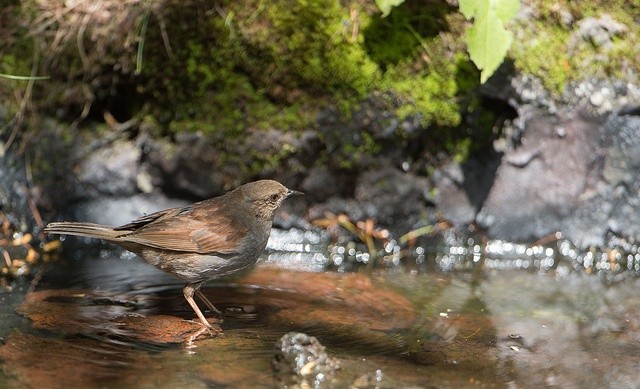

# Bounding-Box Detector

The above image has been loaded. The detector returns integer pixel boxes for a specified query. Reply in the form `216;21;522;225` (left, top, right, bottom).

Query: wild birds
43;178;305;338
